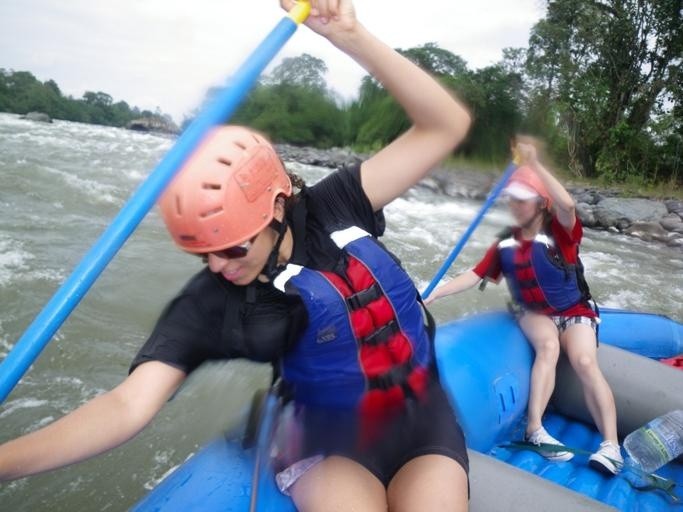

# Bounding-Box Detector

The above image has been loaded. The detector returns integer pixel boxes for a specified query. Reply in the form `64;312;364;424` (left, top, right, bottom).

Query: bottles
623;409;683;476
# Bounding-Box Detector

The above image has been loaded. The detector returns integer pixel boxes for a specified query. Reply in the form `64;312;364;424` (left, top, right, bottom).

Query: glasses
202;236;257;263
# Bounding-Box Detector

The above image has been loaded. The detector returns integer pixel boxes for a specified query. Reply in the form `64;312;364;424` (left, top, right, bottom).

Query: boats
127;307;683;511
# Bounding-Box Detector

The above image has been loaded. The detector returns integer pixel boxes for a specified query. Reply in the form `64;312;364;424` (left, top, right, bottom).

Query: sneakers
523;426;574;462
589;441;624;476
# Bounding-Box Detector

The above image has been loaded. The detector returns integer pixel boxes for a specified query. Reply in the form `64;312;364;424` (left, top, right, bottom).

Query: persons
0;1;474;511
411;130;625;479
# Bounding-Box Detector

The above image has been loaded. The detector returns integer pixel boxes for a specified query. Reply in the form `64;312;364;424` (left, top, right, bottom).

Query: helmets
159;125;293;253
500;165;552;212
